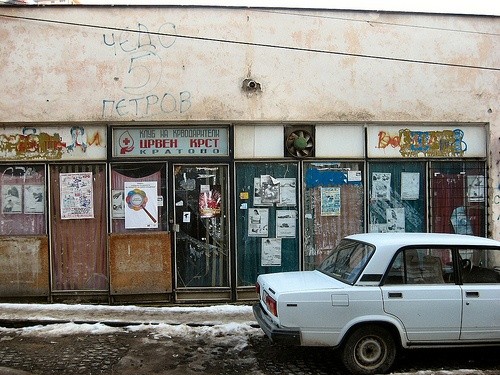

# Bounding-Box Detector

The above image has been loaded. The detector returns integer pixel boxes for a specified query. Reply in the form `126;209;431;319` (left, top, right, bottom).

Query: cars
251;232;499;375
